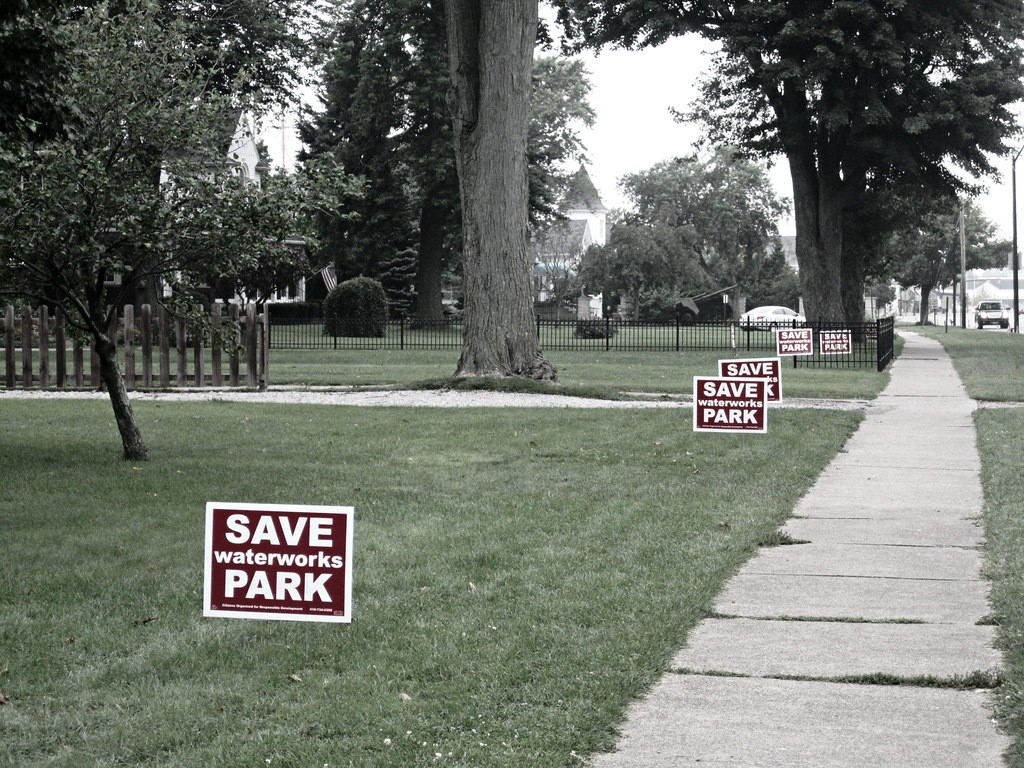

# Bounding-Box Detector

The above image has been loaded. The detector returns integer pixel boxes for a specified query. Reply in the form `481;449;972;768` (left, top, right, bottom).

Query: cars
739;306;806;332
974;301;1010;330
